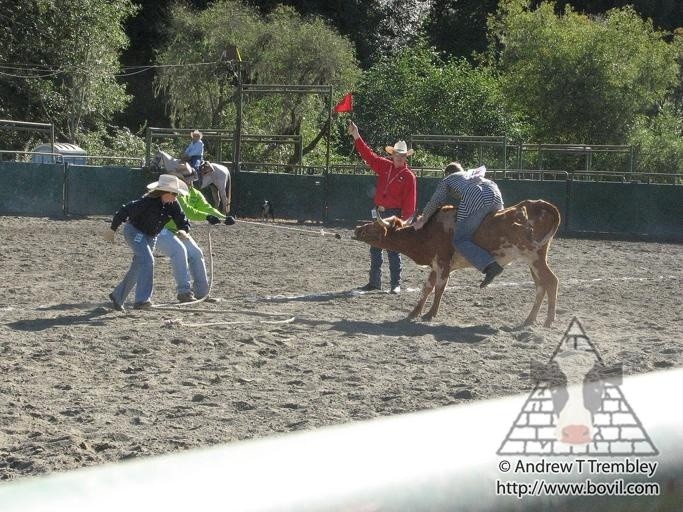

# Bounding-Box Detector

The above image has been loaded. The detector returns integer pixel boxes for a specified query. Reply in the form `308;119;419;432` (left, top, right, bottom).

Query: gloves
206;214;221;225
174;229;187;241
223;215;235;226
101;227;116;245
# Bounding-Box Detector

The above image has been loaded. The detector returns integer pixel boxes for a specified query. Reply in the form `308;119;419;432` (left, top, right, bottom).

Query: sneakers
175;290;197;304
132;301;152;310
107;291;127;315
197;294;219;303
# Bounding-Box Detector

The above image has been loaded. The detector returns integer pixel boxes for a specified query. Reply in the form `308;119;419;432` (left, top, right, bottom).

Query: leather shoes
479;262;504;289
388;285;401;294
356;283;380;291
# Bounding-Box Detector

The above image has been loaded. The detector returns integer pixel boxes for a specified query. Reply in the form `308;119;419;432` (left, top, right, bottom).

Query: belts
375;204;401;212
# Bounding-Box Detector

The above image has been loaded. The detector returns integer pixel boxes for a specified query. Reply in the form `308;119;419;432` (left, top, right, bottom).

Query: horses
148;145;232;216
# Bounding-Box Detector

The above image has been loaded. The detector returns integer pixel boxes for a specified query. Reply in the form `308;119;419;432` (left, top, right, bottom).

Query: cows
530;352;625;447
351;199;561;329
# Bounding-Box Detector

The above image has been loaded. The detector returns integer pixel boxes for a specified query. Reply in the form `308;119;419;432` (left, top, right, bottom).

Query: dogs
260;200;275;223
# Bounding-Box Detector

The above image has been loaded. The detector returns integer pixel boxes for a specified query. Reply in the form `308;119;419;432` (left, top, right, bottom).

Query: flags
331;92;353;112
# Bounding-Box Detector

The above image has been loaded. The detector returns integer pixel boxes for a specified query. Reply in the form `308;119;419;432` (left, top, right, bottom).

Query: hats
383;139;415;157
189;129;202;140
167;161;199;183
145;173;190;197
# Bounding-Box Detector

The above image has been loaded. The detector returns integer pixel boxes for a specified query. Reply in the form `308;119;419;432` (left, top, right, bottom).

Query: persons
181;129;205;189
415;163;504;288
155;163;236;306
108;174;190;311
348;121;417;293
